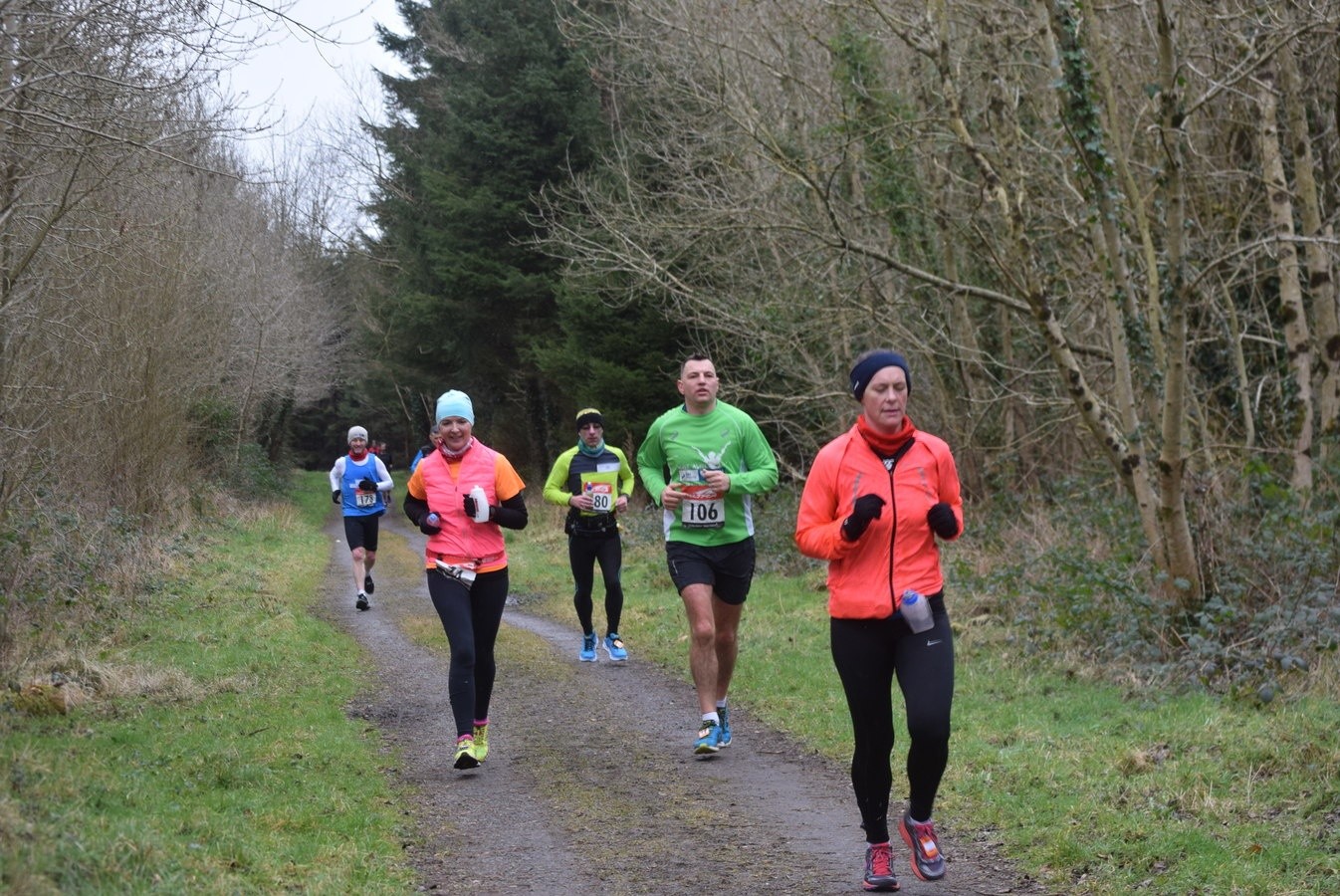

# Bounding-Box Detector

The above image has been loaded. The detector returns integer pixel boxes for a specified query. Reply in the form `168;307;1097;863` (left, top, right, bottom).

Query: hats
436;389;475;430
348;426;369;444
577;408;603;430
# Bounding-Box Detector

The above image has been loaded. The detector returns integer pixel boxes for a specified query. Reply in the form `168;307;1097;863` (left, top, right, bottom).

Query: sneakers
356;595;371;611
898;811;947;881
693;720;722;752
365;576;375;593
579;632;599;663
863;844;900;891
472;721;490;762
453;742;480;769
715;706;732;748
601;632;628;661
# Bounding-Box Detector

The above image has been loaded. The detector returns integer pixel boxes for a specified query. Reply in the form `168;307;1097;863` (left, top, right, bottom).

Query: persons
542;407;634;661
637;353;780;752
795;349;964;890
403;389;529;770
329;425;394;611
367;425;441;507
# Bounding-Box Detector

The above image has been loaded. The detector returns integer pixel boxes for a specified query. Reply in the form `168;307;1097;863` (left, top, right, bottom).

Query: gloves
843;494;887;540
359;476;378;491
463;494;494;521
420;511;442;535
333;489;342;503
926;504;959;539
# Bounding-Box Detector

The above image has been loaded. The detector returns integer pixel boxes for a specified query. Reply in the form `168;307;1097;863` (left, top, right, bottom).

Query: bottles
427;513;441;528
585;482;593;509
900;587;935;634
